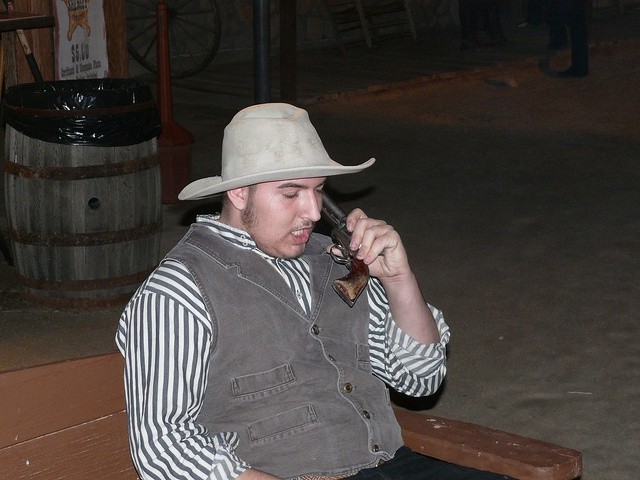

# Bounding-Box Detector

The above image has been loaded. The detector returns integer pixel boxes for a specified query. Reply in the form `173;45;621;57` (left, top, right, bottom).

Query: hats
177;103;376;201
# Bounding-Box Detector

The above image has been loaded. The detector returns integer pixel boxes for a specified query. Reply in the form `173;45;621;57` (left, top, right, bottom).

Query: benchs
0;351;582;479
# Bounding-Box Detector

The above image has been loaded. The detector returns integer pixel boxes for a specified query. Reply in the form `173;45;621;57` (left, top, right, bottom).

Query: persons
115;103;451;480
